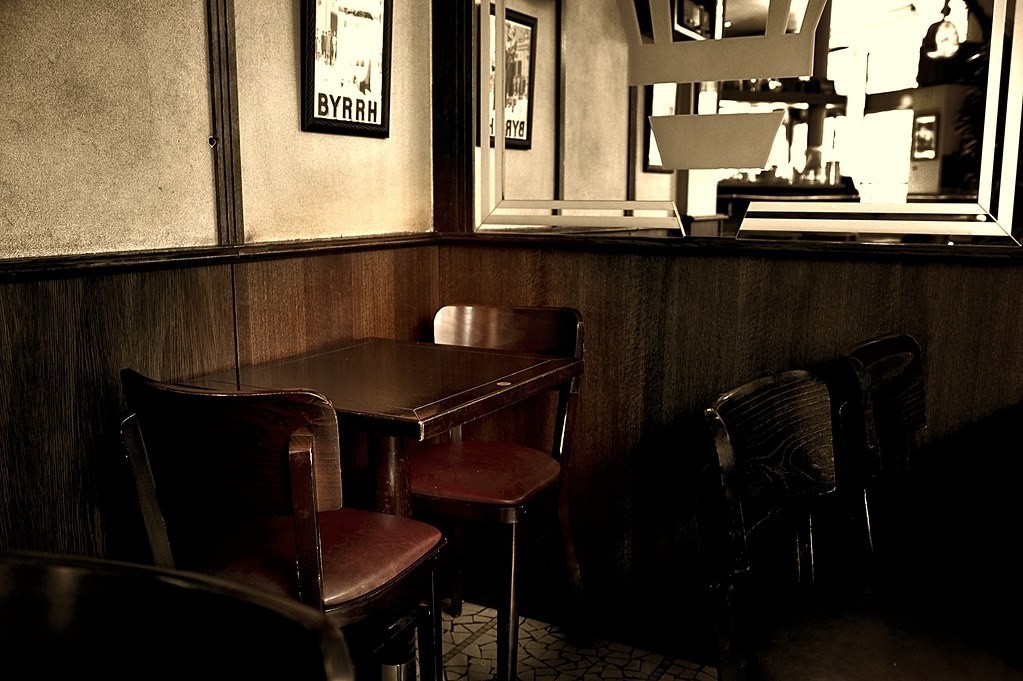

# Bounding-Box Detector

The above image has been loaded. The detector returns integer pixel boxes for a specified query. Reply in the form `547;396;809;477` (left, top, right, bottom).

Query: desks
175;337;586;681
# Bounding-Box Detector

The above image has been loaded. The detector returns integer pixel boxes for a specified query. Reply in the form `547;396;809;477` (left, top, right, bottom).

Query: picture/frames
300;0;393;138
476;2;538;151
910;111;938;161
643;82;679;174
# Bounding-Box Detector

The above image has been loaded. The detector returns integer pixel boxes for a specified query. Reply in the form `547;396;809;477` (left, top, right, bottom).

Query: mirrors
432;0;1023;262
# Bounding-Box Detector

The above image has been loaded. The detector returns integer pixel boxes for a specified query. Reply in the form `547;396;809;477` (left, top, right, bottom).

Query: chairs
0;550;356;681
702;331;1023;681
118;365;450;681
413;305;593;681
